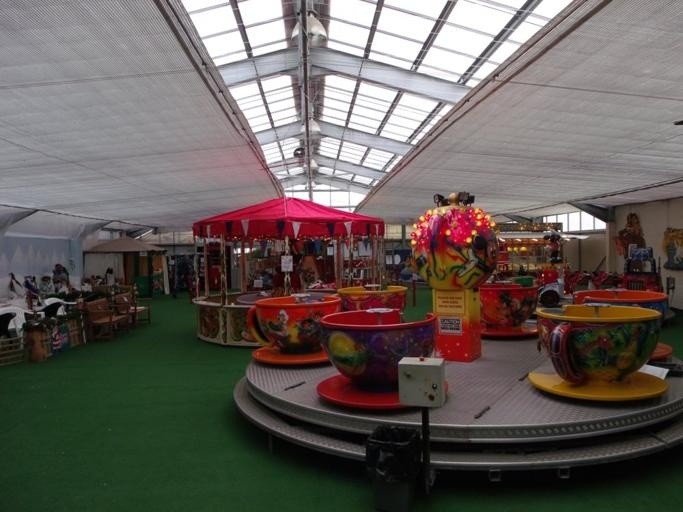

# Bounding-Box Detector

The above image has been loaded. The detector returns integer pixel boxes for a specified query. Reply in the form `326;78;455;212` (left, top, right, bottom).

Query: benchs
85;296;133;342
115;291;151;326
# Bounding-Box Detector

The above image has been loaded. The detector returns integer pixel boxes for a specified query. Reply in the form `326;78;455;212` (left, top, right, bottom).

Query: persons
271;265;283;288
22;262;120;303
618;213;645;257
289;263;301;291
663;226;682;268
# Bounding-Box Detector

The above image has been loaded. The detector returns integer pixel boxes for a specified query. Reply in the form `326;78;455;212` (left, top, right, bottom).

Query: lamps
303;158;318;169
292;0;327;47
306;180;316;188
301;117;320;133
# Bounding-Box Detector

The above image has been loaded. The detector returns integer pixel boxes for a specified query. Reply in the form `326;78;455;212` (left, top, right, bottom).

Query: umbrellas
82;234;167;285
